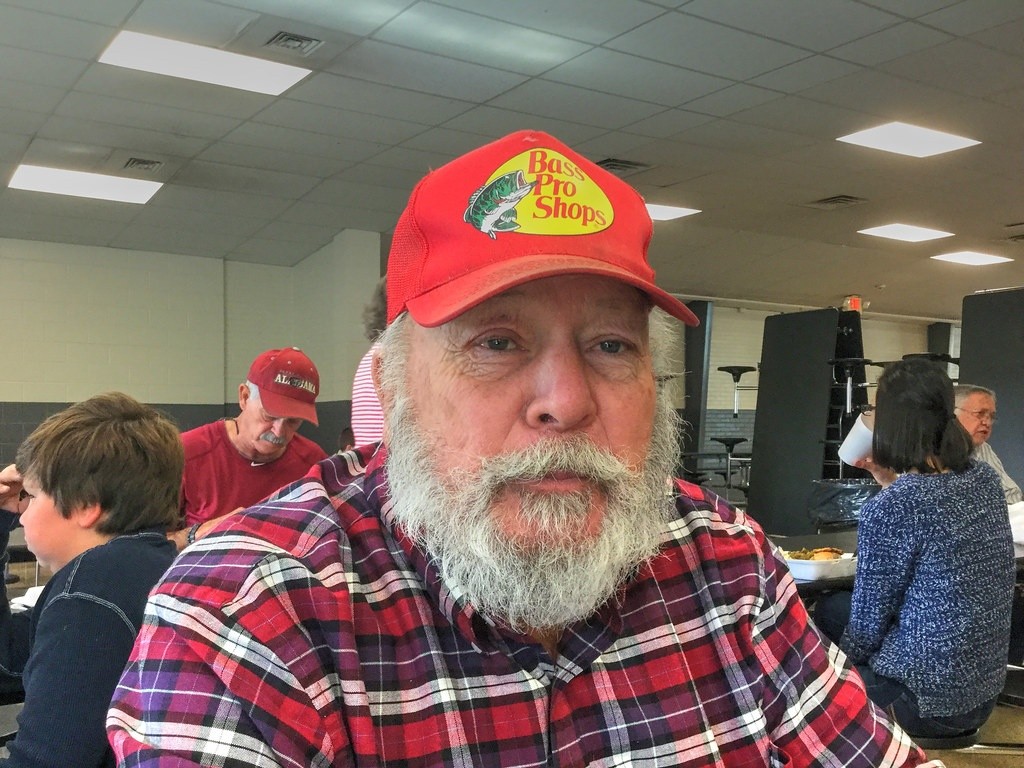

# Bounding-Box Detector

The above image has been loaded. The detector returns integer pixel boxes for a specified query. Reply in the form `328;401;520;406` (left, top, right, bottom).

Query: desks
682;453;752;459
744;287;1024;603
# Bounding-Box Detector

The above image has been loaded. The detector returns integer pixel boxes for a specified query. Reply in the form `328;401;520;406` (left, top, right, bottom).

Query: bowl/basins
777;546;858;580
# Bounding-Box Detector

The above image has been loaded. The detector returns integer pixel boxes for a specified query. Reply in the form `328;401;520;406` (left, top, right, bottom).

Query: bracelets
187;522;203;545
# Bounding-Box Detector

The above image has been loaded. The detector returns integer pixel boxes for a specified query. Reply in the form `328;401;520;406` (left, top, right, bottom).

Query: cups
838;410;875;467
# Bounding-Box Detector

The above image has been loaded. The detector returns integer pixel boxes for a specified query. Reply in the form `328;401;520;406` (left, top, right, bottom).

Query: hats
248;345;323;427
385;129;700;331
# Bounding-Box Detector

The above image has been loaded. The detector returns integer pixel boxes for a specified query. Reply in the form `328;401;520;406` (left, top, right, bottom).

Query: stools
910;664;1024;755
678;352;960;506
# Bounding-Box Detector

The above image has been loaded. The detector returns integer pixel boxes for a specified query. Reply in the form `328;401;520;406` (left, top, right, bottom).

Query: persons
0;391;184;768
351;273;388;449
814;358;1016;738
167;347;329;553
953;384;1023;504
105;129;947;768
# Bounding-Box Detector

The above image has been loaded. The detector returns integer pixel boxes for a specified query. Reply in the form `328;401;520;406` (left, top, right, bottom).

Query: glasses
955;407;999;424
861;404;878;416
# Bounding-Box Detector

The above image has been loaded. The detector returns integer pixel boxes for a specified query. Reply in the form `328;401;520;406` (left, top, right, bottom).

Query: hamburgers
808;547;842;563
